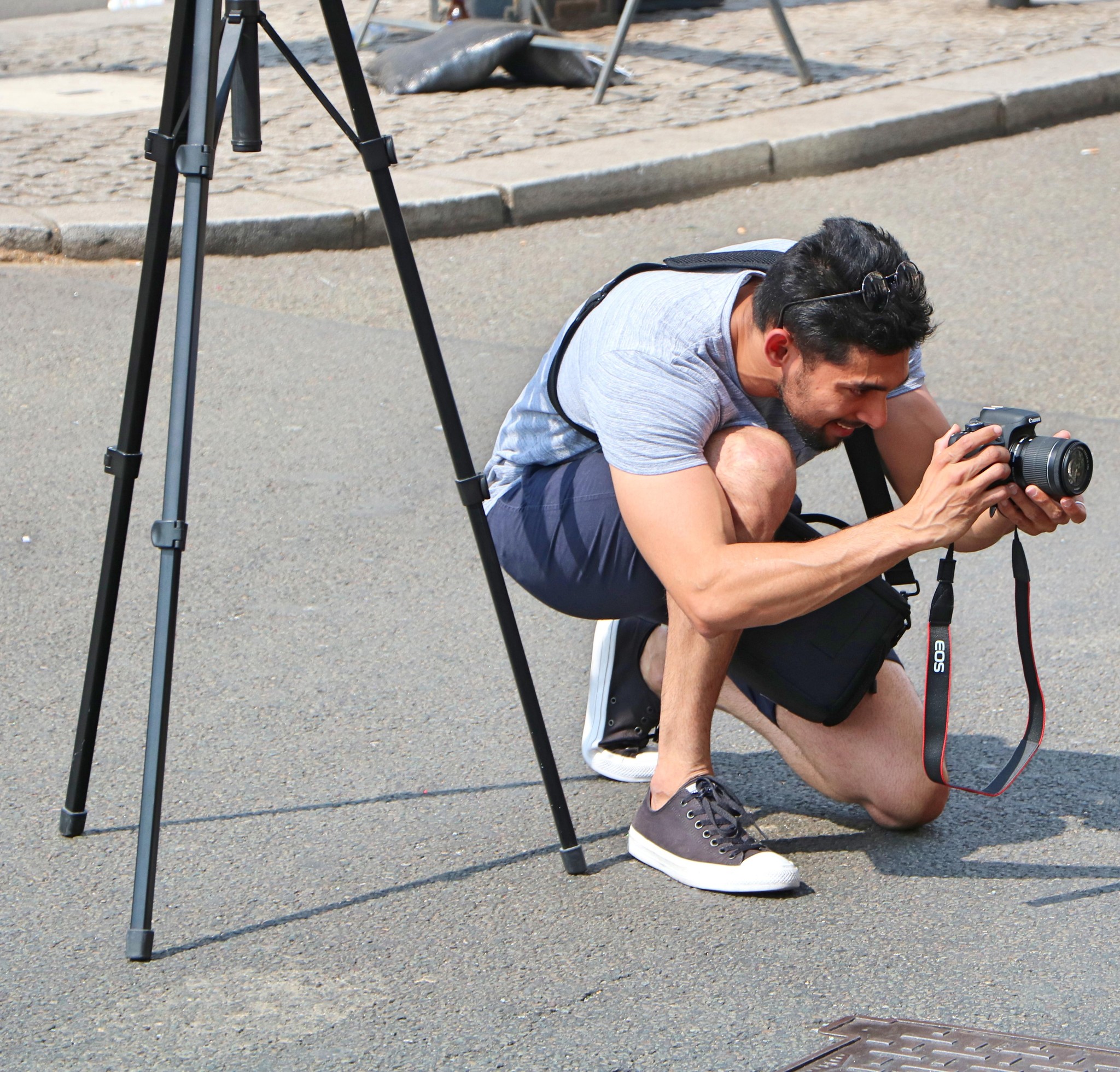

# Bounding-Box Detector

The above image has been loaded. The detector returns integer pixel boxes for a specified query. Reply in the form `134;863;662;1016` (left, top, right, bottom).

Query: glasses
777;262;921;332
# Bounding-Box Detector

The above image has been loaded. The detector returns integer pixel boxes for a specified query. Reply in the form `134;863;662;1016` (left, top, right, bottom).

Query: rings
1075;500;1086;510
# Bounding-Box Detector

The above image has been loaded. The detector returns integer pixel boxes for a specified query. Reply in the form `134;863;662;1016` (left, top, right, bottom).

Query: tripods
58;0;591;961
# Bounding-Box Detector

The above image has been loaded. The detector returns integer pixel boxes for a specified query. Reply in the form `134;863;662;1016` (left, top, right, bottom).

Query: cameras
948;405;1093;503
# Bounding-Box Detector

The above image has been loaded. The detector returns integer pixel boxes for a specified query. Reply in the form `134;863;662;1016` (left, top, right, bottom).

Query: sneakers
582;611;662;780
628;776;799;892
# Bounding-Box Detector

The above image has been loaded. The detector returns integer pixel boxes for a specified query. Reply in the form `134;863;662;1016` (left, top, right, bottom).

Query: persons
478;215;1090;894
